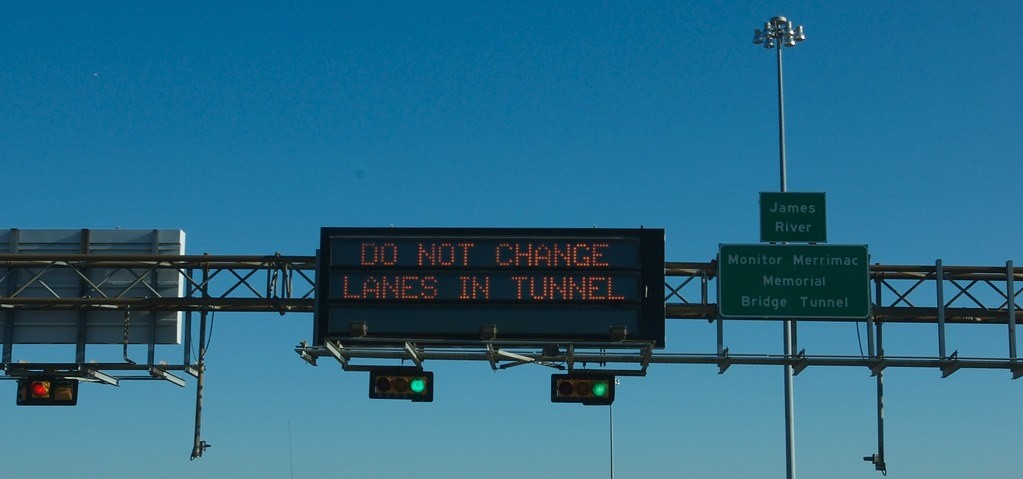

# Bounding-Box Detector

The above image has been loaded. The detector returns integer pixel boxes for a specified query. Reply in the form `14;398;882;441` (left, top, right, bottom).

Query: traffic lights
16;378;78;405
556;378;611;397
373;374;428;396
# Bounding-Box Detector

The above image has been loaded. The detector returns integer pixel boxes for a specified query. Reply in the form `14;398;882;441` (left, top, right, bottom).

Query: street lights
753;13;808;478
